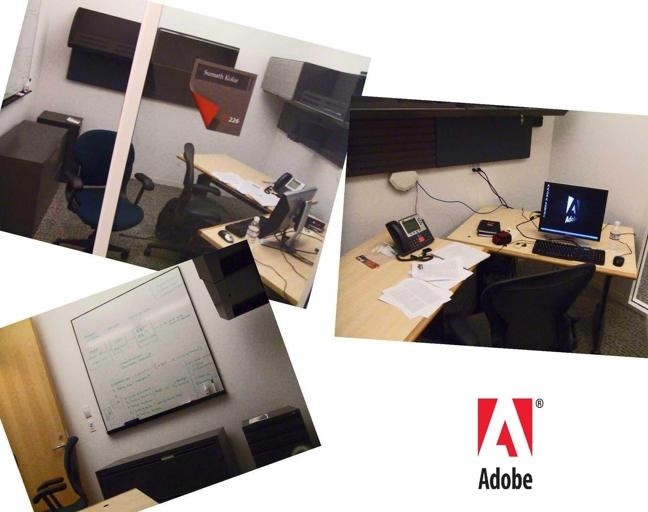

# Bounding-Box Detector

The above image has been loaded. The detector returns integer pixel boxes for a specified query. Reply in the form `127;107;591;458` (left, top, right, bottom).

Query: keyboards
225;215;265;236
533;239;606;265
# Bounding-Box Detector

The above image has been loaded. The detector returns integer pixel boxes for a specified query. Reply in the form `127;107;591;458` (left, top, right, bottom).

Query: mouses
218;230;233;242
612;255;624;267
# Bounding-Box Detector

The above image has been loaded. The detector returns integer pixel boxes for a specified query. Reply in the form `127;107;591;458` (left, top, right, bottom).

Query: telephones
385;214;434;257
272;172;306;195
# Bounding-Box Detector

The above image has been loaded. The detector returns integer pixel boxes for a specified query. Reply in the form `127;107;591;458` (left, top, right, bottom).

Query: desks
173;150;327;307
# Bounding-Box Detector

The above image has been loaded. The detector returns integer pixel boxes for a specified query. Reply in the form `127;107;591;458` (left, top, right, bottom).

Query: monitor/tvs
538;181;608;247
258;187;319;264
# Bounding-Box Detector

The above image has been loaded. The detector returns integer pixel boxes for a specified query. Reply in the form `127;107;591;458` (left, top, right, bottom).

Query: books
477;219;500;238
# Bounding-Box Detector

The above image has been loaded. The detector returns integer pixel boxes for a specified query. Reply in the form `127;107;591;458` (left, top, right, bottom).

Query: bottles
607;221;622;255
245;217;260;251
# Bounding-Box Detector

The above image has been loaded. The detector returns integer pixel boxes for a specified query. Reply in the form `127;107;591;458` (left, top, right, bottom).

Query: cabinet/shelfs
95;406;314;504
0;109;84;238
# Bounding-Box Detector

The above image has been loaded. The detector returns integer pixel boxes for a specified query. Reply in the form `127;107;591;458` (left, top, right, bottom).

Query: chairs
30;434;90;512
145;142;228;266
52;127;155;262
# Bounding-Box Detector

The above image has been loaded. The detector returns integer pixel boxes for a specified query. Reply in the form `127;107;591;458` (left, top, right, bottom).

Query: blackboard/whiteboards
70;266;226;435
0;0;42;108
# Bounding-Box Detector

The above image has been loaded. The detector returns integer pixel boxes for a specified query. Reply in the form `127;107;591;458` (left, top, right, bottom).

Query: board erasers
125;418;139;426
